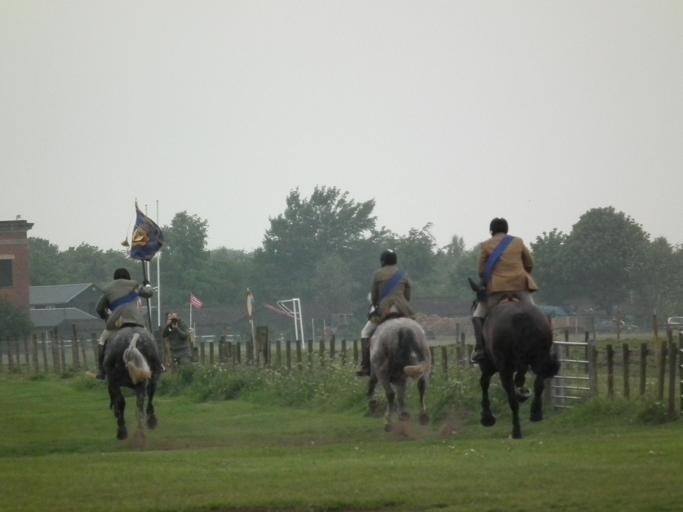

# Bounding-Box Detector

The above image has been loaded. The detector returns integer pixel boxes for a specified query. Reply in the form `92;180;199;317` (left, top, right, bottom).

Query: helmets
114;268;130;280
490;218;508;233
380;249;397;264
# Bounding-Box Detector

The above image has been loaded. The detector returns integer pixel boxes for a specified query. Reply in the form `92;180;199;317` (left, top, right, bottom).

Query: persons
95;266;166;382
162;311;193;382
469;217;539;367
351;248;415;376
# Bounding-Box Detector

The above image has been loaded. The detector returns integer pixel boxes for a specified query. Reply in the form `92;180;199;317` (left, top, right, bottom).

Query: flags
191;295;202;310
129;211;165;261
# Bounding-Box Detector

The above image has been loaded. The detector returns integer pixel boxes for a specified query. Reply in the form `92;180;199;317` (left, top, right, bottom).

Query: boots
355;338;370;376
471;314;486;363
95;344;105;379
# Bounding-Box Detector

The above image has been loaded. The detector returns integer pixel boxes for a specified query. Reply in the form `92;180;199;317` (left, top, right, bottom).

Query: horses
362;292;432;433
102;325;158;440
467;276;561;439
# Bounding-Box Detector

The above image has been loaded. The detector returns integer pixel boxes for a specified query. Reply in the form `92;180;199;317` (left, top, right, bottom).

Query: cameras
171;318;177;325
